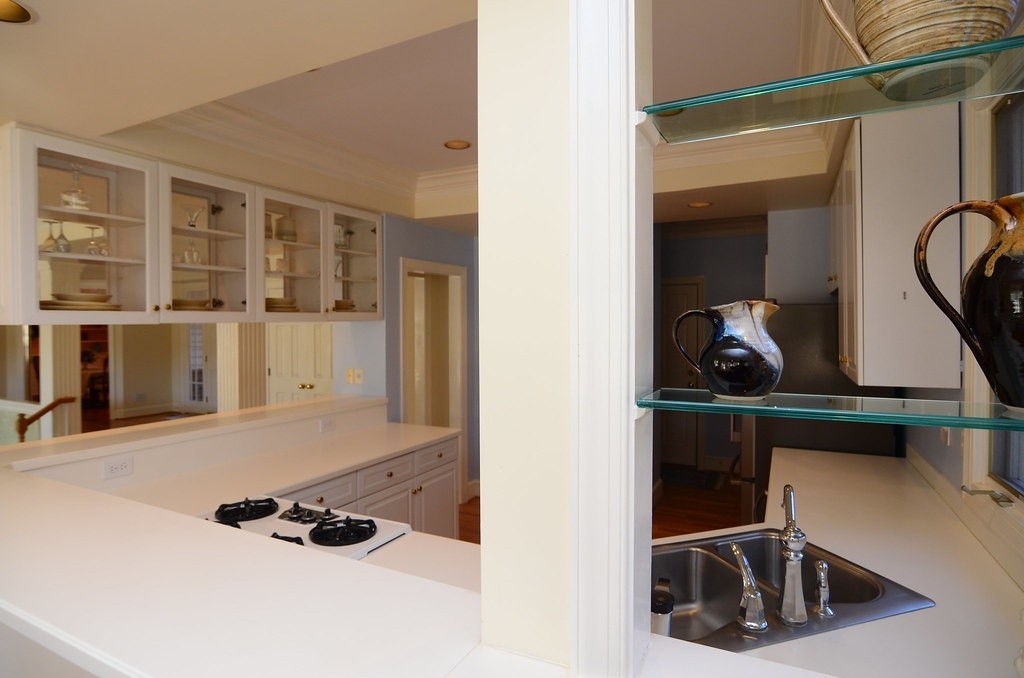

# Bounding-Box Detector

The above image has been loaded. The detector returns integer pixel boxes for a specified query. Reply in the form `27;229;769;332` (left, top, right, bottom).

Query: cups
180;204;206;226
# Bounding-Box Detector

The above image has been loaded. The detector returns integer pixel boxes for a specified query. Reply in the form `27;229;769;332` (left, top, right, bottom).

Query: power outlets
354;368;365;385
345;368;353;384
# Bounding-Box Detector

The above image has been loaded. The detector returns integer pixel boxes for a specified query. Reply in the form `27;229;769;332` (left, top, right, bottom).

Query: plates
40;301;122;312
335;307;355;309
173;305;214;310
266;305;297;308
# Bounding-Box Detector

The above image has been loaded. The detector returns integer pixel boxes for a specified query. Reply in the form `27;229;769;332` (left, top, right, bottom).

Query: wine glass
86;226;113;256
53;218;71;252
40;219;60;252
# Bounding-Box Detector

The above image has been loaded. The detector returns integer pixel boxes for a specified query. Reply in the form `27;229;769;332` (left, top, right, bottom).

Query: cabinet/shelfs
279;472;357;514
18;123;253;324
357;435;460;539
254;188;383;322
827;101;960;386
637;37;1024;432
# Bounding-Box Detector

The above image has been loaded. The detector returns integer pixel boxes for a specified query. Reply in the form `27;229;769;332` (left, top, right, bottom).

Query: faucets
779;484;807;627
728;541;769;632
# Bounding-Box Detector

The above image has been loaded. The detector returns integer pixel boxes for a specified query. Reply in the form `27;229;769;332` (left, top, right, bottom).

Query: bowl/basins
335;299;353;307
265;298;286;304
173;298;210;306
50;293;113;302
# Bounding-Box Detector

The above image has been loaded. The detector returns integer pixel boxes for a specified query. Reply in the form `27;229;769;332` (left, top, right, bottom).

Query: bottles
819;0;1020;101
184;238;201;263
913;194;1024;413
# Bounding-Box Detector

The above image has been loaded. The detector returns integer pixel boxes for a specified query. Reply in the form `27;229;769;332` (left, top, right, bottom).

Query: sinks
650;548;782;652
711;539;887;607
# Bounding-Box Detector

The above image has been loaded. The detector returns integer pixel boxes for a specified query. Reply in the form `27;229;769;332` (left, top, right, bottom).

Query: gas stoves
194;494;413;560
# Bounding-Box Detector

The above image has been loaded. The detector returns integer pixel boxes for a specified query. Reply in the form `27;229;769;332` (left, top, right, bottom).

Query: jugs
674;299;782;401
